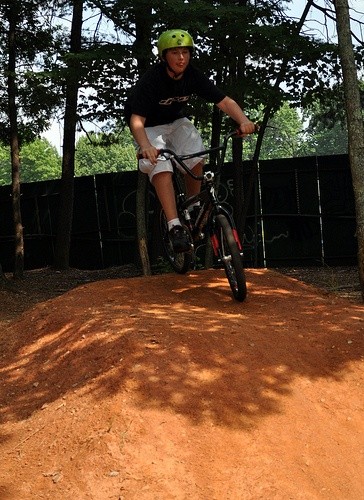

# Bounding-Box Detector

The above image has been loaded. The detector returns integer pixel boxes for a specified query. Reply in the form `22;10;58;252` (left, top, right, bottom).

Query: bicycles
136;126;247;303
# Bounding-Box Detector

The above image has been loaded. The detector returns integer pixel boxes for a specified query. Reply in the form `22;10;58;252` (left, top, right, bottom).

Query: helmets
157;29;194;57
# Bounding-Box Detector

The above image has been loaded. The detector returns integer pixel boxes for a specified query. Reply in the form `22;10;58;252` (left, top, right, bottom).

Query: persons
128;28;256;256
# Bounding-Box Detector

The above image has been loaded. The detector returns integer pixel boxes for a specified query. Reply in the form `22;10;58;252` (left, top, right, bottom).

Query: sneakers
187;205;204;239
169;225;191;252
